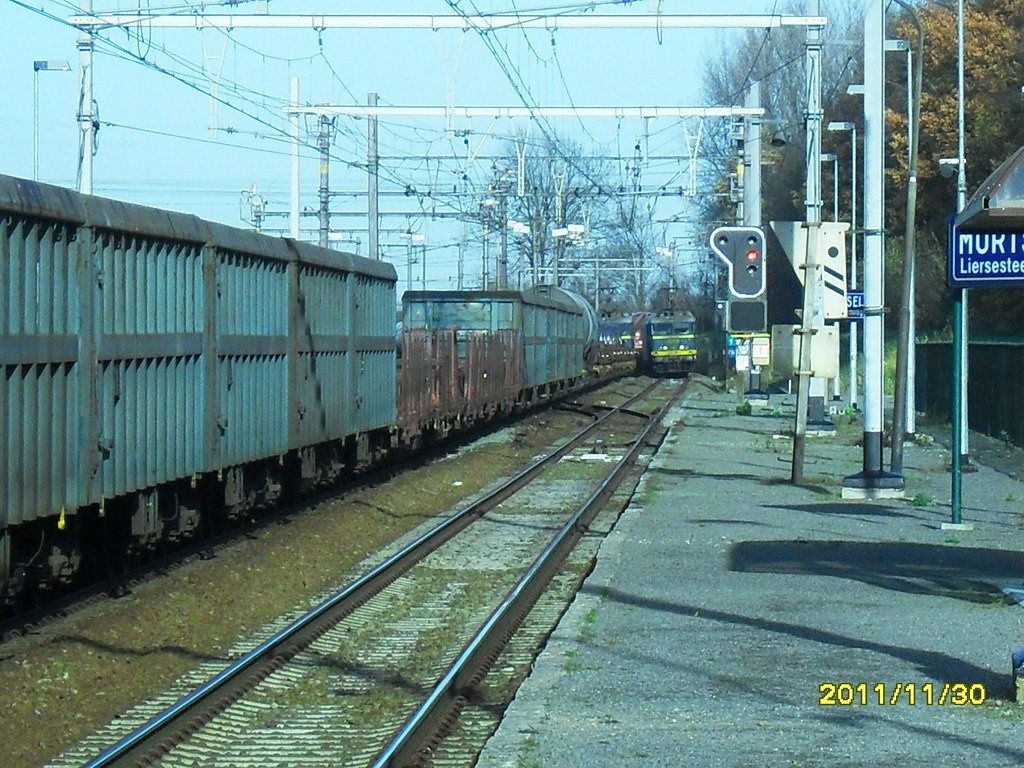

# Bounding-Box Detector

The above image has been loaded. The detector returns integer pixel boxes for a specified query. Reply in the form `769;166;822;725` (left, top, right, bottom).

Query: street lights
821;39;919;442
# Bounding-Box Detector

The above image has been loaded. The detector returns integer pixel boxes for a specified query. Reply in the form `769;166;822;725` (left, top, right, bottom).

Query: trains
0;170;624;626
618;309;699;377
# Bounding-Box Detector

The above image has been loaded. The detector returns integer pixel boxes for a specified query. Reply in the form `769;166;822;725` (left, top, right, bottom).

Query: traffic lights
710;226;767;297
